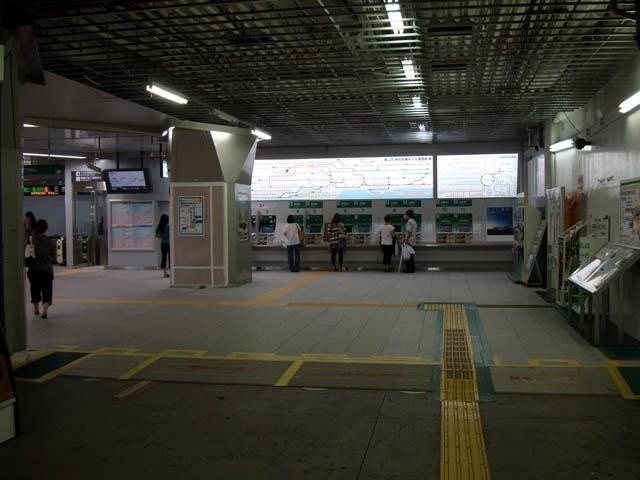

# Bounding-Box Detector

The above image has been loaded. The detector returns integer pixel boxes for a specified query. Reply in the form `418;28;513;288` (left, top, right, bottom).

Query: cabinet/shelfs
511;205;543;277
580;234;608;330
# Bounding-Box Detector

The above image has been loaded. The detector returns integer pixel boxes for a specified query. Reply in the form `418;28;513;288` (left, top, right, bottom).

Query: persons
379;215;396;272
155;214;170;277
189;220;202;233
283;215;301;272
401;209;417;273
97;216;104;236
327;213;347;271
26;219;57;320
22;211;35;278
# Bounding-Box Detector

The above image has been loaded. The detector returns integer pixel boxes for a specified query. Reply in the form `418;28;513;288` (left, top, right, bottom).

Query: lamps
618;87;640;117
548;137;588;155
146;79;188;105
251;126;272;139
214;108;238;124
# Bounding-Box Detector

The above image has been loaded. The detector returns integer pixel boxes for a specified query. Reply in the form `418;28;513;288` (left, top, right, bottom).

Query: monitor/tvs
393;224;419;232
440;224;471;232
259;224;275;233
102;168;152;194
344;224;369;233
300;225;321;233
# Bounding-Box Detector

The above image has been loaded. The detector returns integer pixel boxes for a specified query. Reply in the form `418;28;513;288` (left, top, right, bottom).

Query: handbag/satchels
404;246;415;260
25;245;36;258
395;240;398;256
339;234;349;241
299;231;305;241
